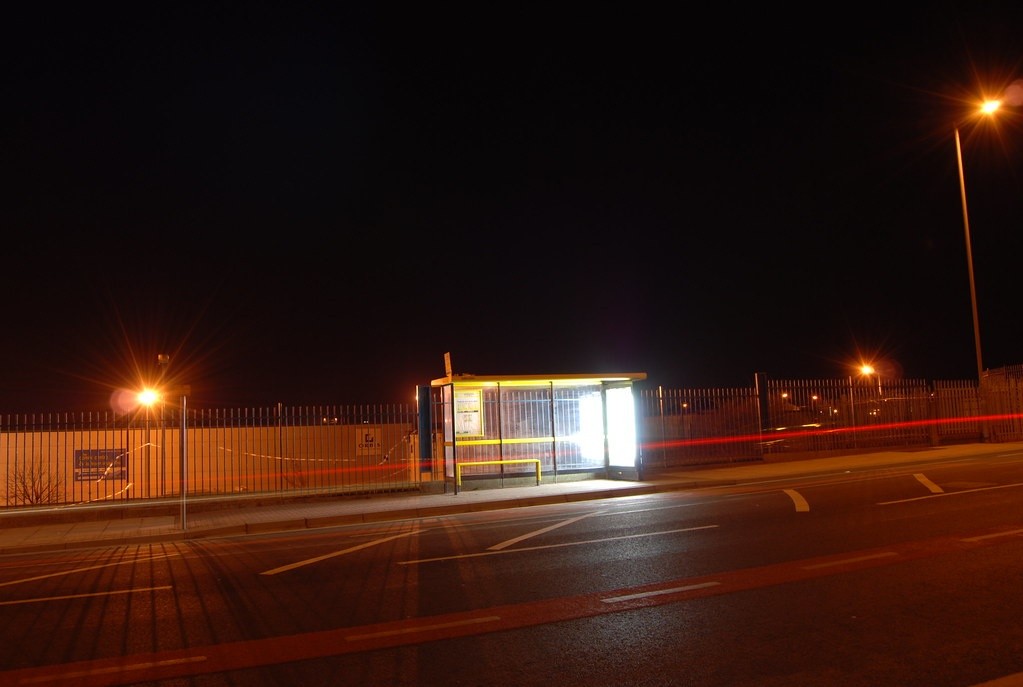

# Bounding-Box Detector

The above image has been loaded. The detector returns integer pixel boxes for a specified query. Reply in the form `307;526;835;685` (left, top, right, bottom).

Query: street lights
951;99;1002;383
